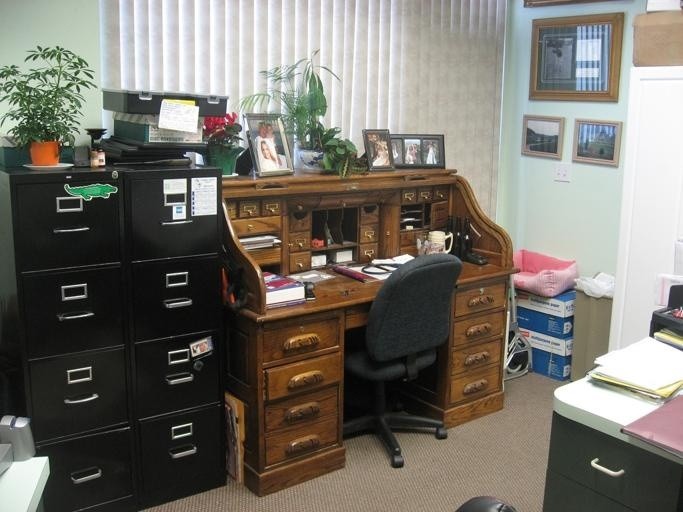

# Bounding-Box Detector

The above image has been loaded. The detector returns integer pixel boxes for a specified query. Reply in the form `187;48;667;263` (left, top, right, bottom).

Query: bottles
89;146;97;168
97;147;104;168
446;215;472;260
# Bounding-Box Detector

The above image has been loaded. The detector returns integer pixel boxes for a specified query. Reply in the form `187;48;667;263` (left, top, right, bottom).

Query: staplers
303;281;316;300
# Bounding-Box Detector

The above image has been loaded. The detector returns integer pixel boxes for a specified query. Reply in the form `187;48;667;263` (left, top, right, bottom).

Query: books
618;393;683;460
262;272;305;304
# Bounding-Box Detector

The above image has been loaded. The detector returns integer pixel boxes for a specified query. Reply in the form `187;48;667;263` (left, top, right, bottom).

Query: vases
197;144;244;177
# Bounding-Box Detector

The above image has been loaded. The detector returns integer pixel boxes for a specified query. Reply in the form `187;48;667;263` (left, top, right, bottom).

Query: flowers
201;110;243;147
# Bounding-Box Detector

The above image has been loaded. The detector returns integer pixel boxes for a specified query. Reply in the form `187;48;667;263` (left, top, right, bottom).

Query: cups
428;230;452;255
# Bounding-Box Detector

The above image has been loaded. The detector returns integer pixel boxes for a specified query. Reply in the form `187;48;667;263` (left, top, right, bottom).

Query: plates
21;162;74;172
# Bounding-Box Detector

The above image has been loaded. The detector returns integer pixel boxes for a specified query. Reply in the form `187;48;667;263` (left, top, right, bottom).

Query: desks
0;454;50;512
538;283;681;510
216;168;513;497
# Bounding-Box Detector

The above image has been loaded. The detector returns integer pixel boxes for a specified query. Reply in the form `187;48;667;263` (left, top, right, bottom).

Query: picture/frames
391;134;445;169
360;129;396;172
520;114;566;161
243;111;294;177
526;10;626;105
570;118;623;169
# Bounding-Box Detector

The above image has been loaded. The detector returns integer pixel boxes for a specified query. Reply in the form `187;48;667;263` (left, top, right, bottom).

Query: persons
423;141;439;164
254;122;287;172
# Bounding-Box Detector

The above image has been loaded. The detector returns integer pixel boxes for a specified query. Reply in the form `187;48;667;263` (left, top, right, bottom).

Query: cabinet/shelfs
1;168;233;512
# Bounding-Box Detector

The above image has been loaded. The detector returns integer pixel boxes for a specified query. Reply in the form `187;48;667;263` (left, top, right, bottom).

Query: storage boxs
516;325;571;382
514;286;576;340
111;111;204;145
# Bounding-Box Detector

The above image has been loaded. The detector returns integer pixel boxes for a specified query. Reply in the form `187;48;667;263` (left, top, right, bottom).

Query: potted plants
0;44;99;169
239;47;358;177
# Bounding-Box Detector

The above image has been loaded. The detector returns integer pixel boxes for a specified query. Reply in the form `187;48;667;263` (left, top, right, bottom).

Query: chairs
342;254;463;468
